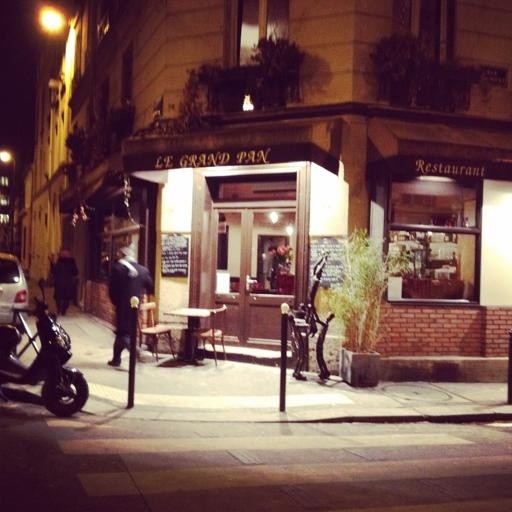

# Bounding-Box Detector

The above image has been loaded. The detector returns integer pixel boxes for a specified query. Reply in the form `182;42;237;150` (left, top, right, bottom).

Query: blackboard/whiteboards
307;235;347;303
161;233;188;278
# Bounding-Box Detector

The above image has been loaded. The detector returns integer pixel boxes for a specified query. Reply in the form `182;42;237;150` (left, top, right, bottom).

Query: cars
0;253;29;334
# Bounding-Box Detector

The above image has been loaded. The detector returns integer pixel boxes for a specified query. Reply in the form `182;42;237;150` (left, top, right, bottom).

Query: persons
52;249;80;315
108;247;153;366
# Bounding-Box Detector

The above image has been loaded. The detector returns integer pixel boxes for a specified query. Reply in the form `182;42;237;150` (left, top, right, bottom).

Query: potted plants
324;224;411;388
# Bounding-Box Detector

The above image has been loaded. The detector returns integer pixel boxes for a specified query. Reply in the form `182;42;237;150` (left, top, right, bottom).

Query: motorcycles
0;276;88;416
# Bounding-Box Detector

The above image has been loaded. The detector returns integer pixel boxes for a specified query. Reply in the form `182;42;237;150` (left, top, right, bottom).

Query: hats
120;246;132;256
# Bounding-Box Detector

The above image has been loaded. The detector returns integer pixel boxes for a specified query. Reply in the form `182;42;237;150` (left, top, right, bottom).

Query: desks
161;308;216;364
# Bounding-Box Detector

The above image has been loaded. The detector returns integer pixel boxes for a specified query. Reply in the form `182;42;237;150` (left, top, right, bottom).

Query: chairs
137;301;177;364
186;303;228;366
